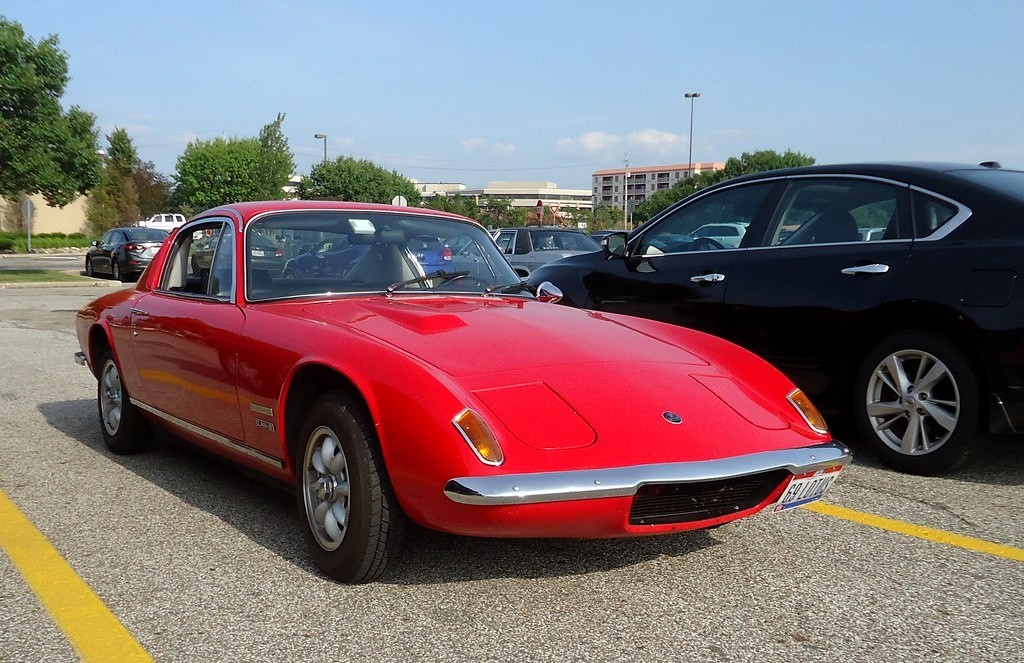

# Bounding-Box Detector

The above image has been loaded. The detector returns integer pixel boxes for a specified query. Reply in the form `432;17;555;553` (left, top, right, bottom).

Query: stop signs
536;200;544;222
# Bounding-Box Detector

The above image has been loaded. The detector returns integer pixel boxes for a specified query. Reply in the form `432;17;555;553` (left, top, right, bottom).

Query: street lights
315;135;328;161
684;93;700;177
623;154;631;230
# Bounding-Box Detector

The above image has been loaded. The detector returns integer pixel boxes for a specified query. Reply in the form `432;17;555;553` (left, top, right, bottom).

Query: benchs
184;269;363;298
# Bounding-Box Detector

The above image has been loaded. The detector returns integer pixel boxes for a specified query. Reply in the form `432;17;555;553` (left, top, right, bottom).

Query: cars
453;228;604;288
525;162;1024;476
563;230;669;255
282;231;460;288
85;227;171;279
858;229;886;241
191;230;285;276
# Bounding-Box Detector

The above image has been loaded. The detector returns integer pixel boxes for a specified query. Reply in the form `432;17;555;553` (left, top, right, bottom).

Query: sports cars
72;200;853;587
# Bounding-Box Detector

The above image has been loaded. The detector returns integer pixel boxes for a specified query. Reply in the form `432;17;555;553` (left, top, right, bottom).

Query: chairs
780;207;858;245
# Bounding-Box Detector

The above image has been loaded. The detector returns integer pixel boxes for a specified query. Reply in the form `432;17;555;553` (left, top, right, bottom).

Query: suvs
136;213;188;235
682;222;749;251
